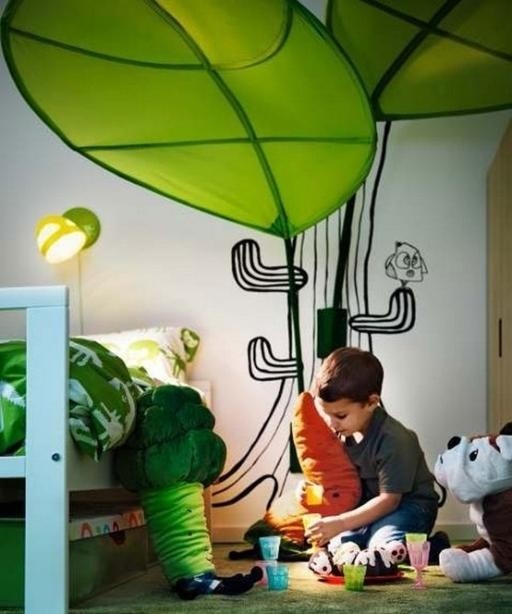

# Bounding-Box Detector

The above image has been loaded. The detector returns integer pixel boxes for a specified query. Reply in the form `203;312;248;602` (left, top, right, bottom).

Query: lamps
34;206;100;263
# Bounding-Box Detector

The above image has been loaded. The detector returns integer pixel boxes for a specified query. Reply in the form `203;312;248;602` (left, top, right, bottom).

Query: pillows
76;326;201;388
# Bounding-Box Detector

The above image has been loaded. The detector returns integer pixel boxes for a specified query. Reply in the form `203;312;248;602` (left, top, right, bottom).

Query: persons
299;347;452;566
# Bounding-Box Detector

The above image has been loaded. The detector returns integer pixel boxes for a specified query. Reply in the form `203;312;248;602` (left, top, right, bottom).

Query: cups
342;564;368;591
255;560;290;592
259;535;282;561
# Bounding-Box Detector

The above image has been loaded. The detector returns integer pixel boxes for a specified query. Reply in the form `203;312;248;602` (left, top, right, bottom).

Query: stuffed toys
112;384;512;600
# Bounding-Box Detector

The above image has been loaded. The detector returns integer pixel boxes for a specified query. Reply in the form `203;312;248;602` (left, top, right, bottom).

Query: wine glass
405;533;431;591
303;513;326;555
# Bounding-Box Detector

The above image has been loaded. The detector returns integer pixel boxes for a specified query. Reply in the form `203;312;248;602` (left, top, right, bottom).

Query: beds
0;284;211;613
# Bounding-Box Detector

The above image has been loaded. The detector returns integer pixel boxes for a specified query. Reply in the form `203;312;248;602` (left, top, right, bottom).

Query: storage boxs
0;499;150;608
